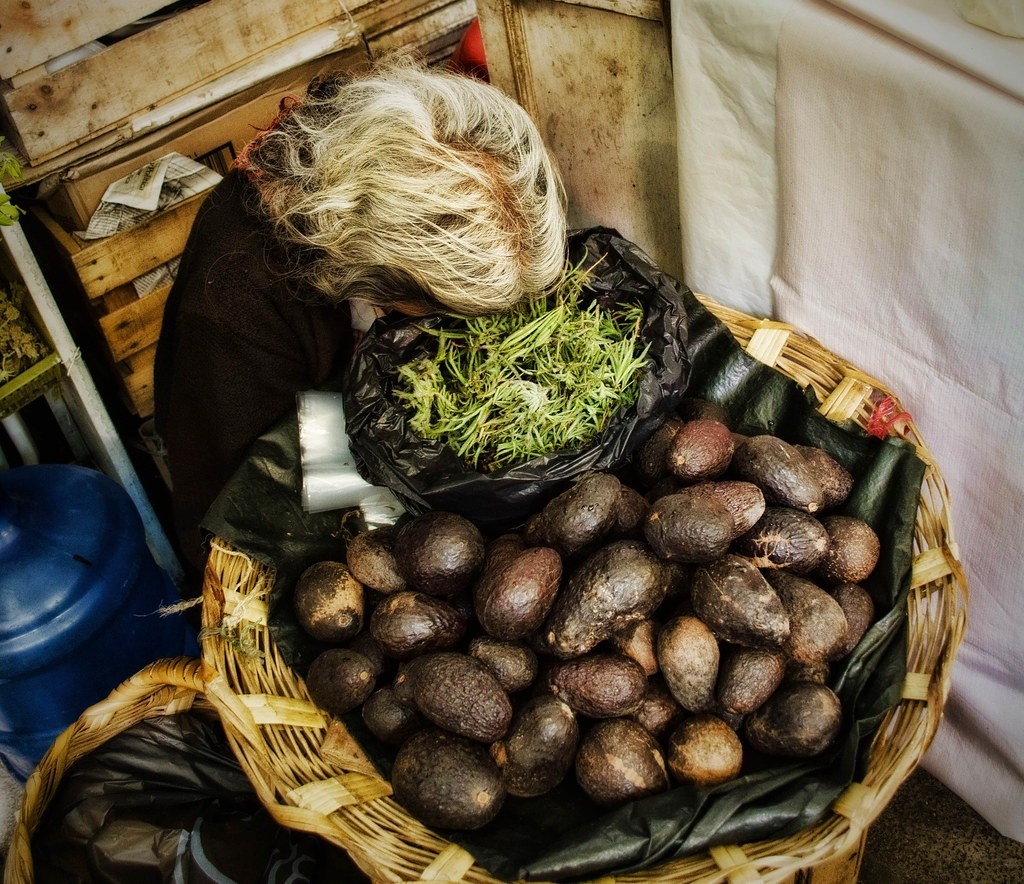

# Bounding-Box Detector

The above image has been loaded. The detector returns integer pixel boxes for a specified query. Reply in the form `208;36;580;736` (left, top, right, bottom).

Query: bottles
1;461;194;779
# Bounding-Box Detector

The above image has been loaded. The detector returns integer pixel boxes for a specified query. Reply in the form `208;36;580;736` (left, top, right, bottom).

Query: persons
151;55;568;525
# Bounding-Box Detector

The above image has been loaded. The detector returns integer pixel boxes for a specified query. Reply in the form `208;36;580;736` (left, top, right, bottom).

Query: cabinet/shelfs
0;181;190;591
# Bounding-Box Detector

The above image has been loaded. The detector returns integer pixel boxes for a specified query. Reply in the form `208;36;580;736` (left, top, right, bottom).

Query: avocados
294;396;880;828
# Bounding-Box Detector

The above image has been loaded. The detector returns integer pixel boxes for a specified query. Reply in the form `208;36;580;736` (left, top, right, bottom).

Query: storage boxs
0;0;474;500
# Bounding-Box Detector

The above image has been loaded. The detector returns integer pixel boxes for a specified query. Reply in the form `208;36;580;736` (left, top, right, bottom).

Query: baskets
0;283;970;884
